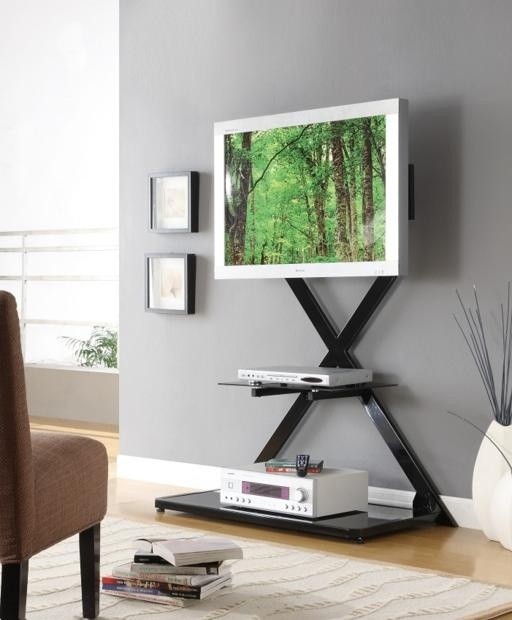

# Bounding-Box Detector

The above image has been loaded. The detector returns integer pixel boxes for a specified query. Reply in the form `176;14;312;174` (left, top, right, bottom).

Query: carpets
0;511;512;620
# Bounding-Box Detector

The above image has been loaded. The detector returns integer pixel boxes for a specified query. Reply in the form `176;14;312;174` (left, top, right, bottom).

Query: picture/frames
142;170;198;314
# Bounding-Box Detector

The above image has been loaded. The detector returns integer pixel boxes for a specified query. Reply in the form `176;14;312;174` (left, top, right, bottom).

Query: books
100;537;244;607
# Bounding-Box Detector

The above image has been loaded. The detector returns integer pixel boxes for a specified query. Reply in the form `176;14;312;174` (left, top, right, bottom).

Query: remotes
296;454;309;477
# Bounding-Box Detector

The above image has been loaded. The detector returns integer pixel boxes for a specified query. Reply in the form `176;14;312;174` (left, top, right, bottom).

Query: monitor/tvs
213;97;409;280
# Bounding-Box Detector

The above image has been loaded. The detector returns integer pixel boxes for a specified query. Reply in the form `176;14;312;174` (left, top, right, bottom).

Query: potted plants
445;280;512;553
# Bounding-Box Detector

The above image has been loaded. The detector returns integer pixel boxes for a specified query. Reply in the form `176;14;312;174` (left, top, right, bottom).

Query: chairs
1;288;108;620
